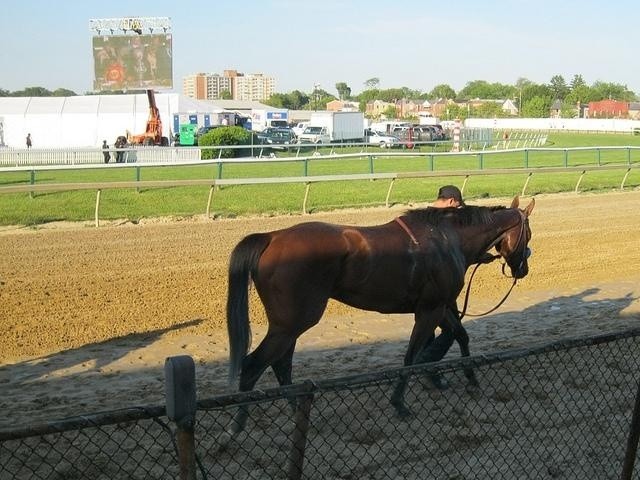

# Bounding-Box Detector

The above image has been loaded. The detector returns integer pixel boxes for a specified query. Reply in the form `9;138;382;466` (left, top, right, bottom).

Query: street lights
314;81;322;111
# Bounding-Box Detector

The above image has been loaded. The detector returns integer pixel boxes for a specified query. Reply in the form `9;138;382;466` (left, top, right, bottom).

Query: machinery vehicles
125;90;169;146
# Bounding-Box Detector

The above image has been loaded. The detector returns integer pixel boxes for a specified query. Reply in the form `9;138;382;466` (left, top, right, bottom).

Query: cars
173;108;310;154
364;121;444;149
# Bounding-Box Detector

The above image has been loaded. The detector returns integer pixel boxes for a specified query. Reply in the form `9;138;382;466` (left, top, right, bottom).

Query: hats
438;185;466;207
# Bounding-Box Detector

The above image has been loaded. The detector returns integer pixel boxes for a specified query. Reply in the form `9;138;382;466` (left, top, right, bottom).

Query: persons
415;184;467;392
101;140;111;163
221;116;229;125
26;133;33;148
115;137;124;163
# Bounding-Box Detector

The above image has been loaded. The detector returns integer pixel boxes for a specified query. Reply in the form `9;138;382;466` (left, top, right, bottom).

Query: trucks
300;110;366;148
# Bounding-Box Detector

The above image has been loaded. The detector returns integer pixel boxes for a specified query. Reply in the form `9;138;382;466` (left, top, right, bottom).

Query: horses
204;195;536;456
